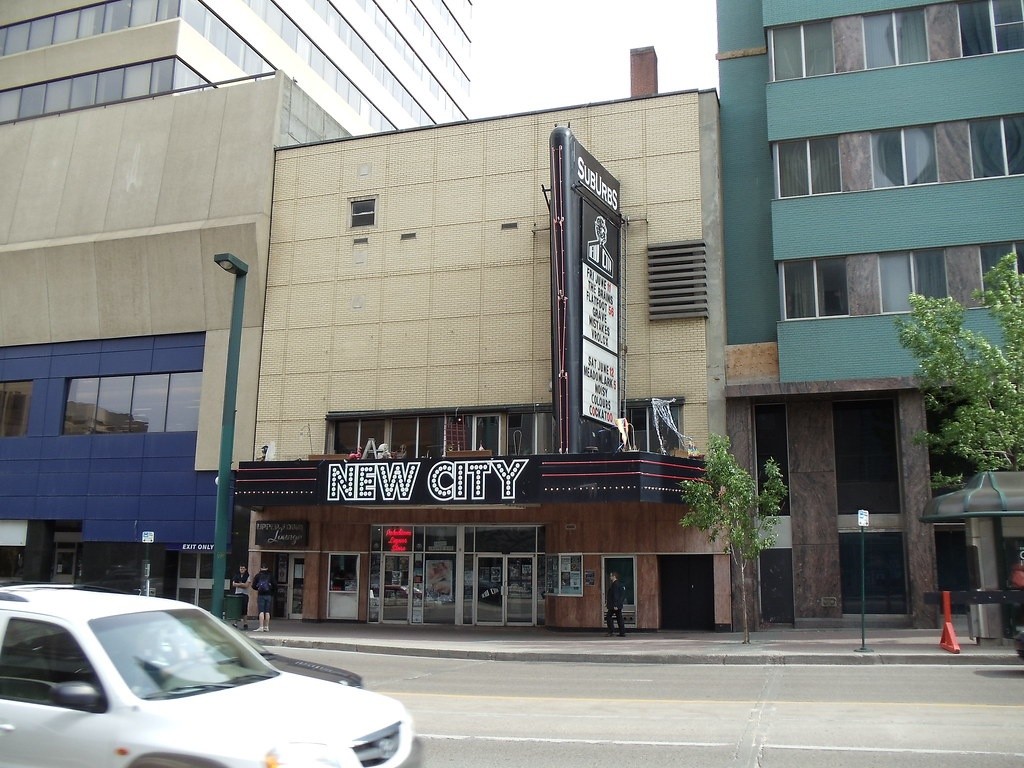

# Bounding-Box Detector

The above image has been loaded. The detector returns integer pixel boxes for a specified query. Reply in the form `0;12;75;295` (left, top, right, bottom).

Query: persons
231;564;252;630
252;562;275;632
603;570;628;637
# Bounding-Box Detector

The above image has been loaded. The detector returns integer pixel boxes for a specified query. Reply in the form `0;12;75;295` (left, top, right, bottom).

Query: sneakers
232;623;237;629
243;623;248;630
260;625;269;632
252;627;263;632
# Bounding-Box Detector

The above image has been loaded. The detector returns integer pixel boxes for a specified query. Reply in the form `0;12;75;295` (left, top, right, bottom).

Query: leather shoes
603;631;614;637
616;632;626;637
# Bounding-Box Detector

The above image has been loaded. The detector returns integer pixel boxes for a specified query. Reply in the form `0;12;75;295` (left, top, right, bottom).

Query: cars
0;588;416;767
12;584;363;688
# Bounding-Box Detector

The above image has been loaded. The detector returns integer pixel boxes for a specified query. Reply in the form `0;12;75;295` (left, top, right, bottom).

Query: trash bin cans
225;595;243;620
968;591;1003;646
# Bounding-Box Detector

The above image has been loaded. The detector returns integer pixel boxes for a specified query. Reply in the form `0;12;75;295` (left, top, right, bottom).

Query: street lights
211;252;249;616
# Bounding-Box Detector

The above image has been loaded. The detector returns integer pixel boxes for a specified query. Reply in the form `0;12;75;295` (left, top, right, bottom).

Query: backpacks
257;571;271;591
